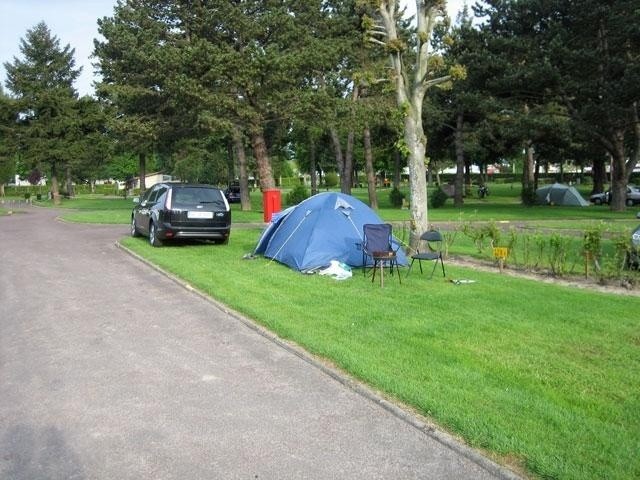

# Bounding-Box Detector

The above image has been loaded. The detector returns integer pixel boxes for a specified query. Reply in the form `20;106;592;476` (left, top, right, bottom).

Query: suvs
130;180;233;247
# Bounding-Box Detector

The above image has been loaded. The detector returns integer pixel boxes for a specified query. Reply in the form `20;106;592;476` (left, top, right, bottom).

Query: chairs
362;224;401;287
406;229;445;279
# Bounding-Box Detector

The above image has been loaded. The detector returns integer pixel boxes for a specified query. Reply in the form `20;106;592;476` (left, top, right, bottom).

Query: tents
251;192;410;273
536;184;589;206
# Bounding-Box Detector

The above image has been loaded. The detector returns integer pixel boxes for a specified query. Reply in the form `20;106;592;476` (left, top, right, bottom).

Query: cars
589;184;640;206
628;208;640;272
224;186;241;202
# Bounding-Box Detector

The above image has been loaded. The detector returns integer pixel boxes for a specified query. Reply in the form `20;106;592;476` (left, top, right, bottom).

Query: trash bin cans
263;189;282;223
